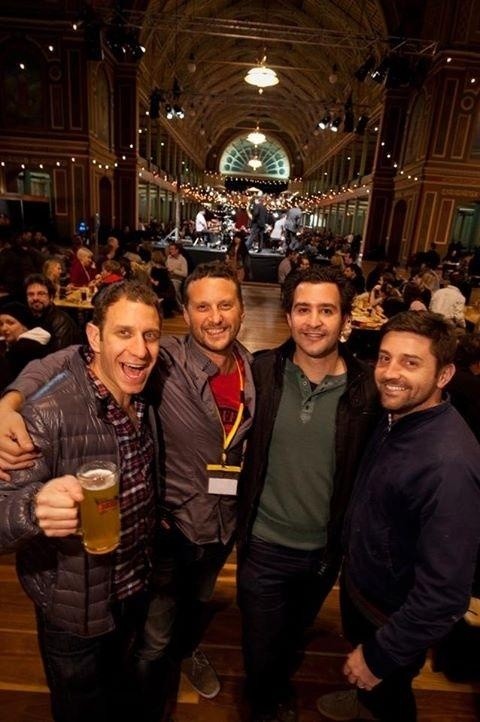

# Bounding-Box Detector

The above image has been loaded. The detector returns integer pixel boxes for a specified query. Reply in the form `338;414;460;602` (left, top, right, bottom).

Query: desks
53;288;95;336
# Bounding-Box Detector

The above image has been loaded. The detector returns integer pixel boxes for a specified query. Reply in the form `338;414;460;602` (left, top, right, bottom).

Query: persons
194;209;215;248
342;252;357;269
0;304;52;393
143;251;176;318
441;250;460;275
38;259;75;300
236;266;375;722
402;272;440;313
226;232;251;286
270;215;286;255
157;219;194;238
222;214;234;230
277;249;295;285
281;259;310;299
246;198;266;253
138;235;158;263
12;231;33;253
284;205;303;251
467;246;480;308
335;310;479;722
0;262;256;699
343;265;366;295
304;228;338;258
369;273;402;309
338;235;356;255
23;274;77;352
100;237;122;261
163;244;188;306
428;277;472;335
69;246;97;288
92;260;124;293
34;233;47;251
0;280;162;722
420;243;440;272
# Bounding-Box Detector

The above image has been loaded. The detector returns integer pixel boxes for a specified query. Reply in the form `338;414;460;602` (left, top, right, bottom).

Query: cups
89;283;95;295
80;288;87;304
73;461;121;555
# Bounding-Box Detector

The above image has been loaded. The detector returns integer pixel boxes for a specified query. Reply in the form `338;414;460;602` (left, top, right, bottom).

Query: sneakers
177;648;220;698
317;689;378;721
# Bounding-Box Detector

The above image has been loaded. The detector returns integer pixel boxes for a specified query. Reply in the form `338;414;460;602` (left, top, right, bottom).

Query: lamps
164;104;175;120
330;118;340;132
247;155;262;171
244;52;278;88
318;115;331;130
247;127;266;146
176;107;184;120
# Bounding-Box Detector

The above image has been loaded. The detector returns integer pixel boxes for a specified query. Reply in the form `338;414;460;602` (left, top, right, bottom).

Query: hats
233;231;247;240
1;303;35;329
